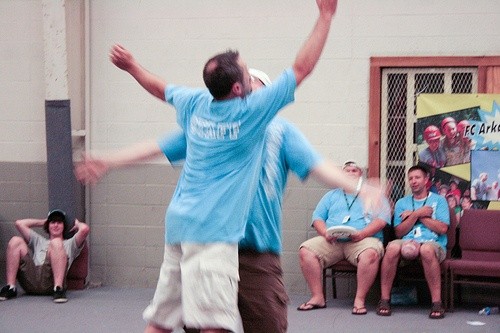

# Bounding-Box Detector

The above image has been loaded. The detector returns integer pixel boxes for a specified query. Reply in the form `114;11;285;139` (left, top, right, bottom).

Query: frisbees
328;226;356;240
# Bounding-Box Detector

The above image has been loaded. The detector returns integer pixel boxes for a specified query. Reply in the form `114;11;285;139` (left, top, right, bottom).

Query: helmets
441;117;455;127
423;125;441;142
457;119;470;132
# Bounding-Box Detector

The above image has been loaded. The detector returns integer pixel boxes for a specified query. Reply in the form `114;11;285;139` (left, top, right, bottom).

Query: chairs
323;209;500;313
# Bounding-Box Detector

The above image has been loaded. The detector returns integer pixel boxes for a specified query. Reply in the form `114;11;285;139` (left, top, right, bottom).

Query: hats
341;159;361;170
48;208;66;217
247;68;272;87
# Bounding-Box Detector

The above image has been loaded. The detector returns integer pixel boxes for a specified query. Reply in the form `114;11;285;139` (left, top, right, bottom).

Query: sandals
376;298;392;316
429;302;445;319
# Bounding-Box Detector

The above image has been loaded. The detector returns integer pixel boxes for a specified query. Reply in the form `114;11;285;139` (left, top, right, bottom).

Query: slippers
297;298;327;311
352;305;368;315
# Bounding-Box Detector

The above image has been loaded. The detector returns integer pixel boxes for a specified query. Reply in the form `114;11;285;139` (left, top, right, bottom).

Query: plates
326;226;357;241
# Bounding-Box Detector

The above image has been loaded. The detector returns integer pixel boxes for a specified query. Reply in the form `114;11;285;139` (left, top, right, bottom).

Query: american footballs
400;240;419;259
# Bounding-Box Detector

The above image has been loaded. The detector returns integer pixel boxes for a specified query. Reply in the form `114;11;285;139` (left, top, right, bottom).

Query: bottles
479;306;499;315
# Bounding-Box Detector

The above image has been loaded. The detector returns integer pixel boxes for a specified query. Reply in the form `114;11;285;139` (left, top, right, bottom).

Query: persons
0;210;90;302
376;166;450;317
297;161;392;314
418;117;500;226
75;69;385;333
109;0;338;333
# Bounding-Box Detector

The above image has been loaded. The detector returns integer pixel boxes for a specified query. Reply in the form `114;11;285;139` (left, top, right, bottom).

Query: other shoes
0;284;17;300
53;286;68;303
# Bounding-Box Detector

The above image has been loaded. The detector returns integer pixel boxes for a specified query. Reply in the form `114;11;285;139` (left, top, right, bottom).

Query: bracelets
357;178;362;192
